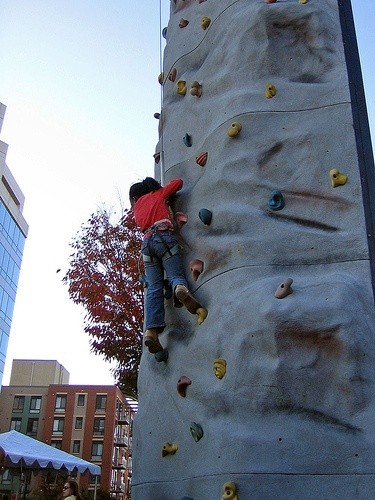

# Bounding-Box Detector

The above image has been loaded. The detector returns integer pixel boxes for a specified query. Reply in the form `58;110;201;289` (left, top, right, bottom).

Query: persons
62;481;81;500
129;177;201;354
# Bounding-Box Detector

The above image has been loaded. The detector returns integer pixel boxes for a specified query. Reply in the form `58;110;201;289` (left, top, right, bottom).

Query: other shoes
176;285;203;314
144;335;162;353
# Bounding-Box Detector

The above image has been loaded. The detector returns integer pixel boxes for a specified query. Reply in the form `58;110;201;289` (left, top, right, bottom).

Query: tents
0;429;101;500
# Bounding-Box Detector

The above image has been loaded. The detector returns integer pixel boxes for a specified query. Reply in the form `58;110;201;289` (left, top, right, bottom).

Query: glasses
63;486;70;490
